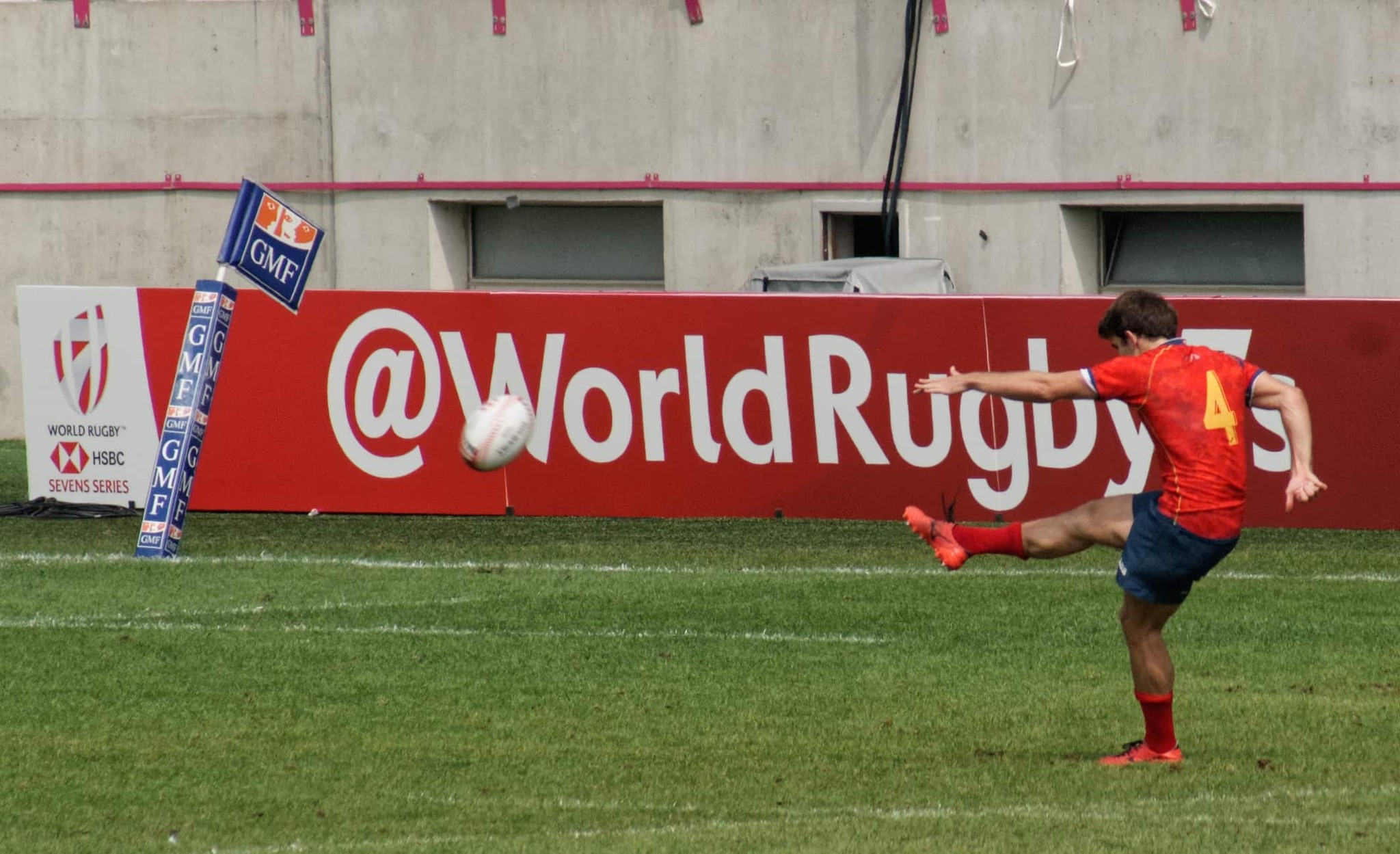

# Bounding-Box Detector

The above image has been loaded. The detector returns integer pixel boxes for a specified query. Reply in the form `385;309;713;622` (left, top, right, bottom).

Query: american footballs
460;395;536;472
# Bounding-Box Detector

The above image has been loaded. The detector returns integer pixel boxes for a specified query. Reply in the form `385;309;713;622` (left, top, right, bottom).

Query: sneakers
1097;739;1184;765
903;506;970;570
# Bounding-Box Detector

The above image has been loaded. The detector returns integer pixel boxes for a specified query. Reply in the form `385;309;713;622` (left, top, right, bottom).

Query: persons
905;290;1328;768
